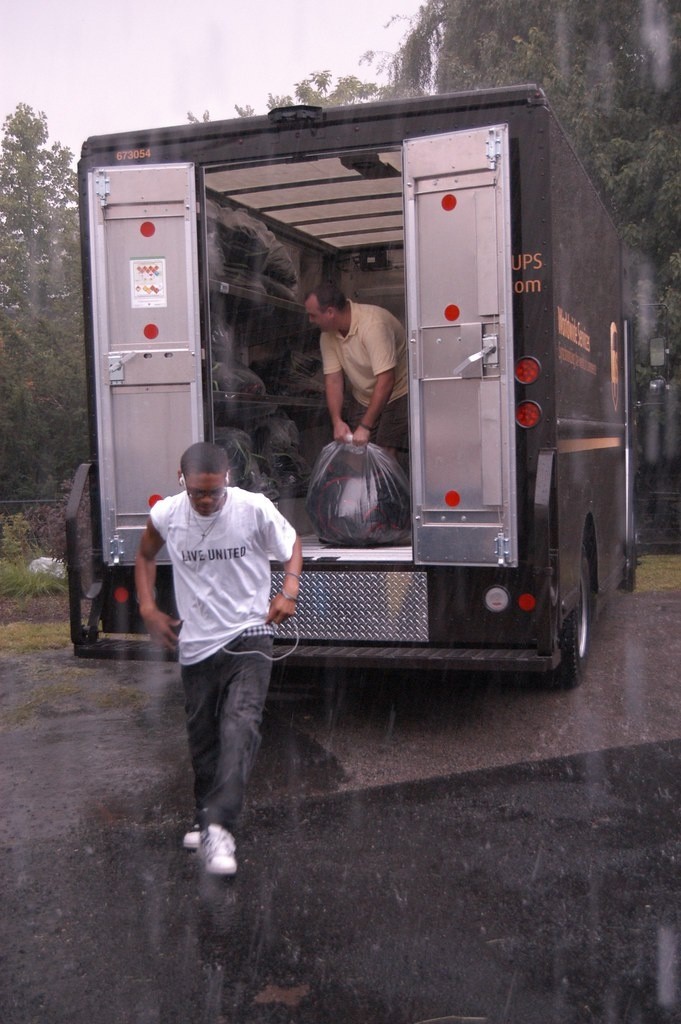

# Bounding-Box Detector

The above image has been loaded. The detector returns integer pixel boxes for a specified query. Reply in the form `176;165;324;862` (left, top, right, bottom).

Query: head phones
179;470;230;489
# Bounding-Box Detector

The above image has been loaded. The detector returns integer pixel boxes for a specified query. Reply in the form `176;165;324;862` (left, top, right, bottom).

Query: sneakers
183;822;204;849
197;824;237;873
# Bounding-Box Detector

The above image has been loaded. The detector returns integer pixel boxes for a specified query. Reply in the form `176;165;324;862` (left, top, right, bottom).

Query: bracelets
360;423;370;430
280;590;299;602
285;573;300;579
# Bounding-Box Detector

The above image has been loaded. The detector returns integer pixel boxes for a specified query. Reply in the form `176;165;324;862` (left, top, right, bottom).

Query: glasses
180;473;229;500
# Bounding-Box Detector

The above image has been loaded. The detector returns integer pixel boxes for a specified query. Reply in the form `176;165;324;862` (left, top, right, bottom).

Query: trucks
63;83;668;690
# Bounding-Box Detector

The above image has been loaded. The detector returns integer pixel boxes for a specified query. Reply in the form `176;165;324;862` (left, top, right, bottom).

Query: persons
304;286;410;459
136;443;303;875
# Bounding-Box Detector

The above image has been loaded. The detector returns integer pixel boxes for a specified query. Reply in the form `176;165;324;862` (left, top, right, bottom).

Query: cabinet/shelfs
200;278;353;410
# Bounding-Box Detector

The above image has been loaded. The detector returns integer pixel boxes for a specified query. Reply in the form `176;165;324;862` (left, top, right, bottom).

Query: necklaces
190;508;222;543
